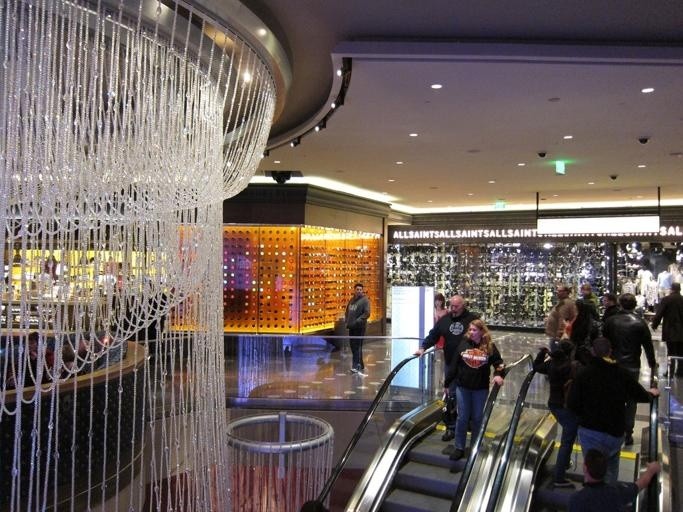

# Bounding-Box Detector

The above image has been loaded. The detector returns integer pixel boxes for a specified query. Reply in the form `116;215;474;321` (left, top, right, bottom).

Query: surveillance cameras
639;138;649;144
272;172;292;184
539;153;546;157
611;175;617;180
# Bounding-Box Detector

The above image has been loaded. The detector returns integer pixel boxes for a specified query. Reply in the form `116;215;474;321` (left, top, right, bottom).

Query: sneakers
441;415;455;441
554;478;572;487
349;368;363;375
449;448;464;460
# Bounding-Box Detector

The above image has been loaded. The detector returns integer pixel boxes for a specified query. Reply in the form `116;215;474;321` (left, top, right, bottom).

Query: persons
281;345;292;373
344;283;371;376
651;281;683;381
11;253;22;266
315;355;336;381
96;261;116;291
411;292;505;459
43;254;58;284
531;280;663;511
